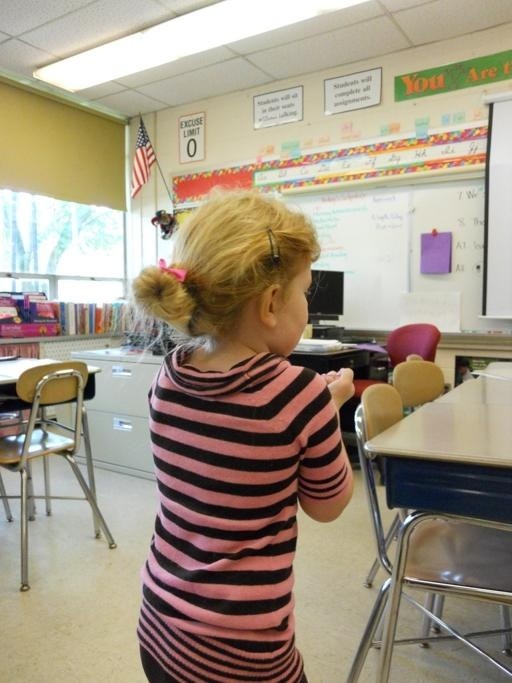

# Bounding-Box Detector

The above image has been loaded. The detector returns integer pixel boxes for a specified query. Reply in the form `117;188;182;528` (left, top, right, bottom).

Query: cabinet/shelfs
278;340;385;464
0;334;164;481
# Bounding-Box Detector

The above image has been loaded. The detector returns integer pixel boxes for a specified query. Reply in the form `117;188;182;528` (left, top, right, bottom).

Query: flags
130;118;158;198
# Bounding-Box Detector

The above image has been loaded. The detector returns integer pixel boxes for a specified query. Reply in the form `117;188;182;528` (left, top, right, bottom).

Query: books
292;338;345;354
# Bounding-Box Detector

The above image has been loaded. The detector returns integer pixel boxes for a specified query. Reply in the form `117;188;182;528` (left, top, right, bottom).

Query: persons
0;290;147;341
132;184;358;682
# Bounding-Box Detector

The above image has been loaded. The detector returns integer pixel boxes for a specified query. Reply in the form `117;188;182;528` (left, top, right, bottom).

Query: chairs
0;361;118;591
362;358;509;595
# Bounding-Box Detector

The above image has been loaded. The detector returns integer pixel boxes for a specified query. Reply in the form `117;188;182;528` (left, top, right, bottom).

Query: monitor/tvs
306;268;344;327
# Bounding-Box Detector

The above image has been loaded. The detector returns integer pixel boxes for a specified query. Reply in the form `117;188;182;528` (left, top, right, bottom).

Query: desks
0;358;101;543
